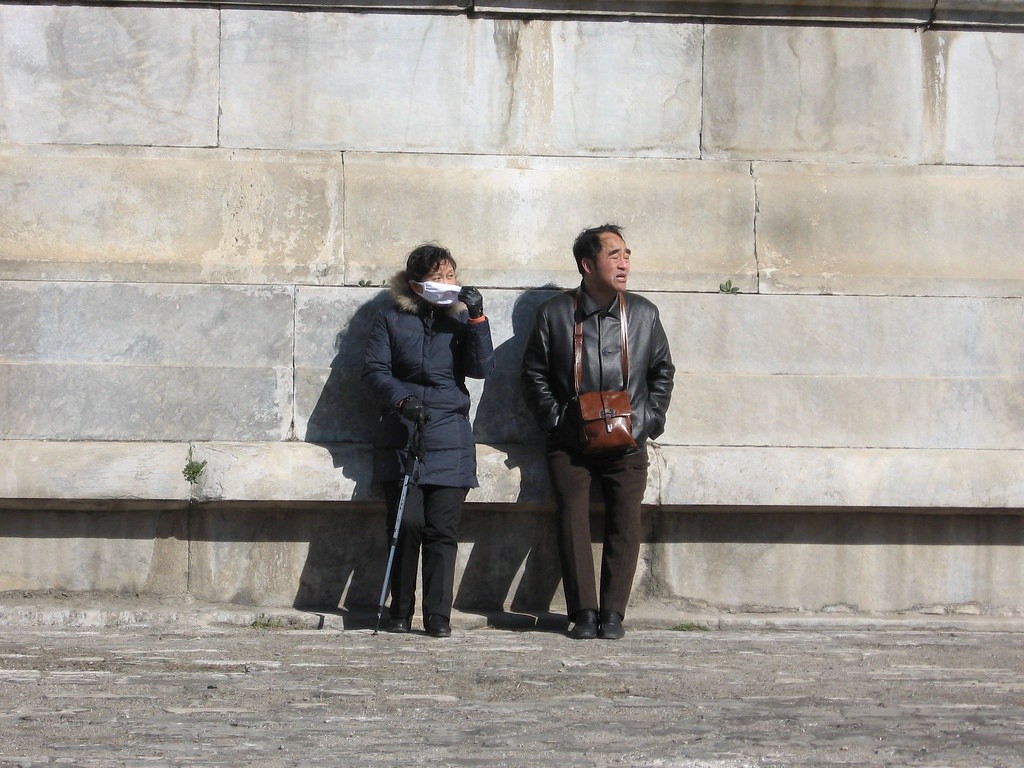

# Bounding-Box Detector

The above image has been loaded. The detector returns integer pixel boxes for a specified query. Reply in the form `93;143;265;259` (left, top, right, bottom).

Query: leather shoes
424;614;451;636
389;615;413;632
598;610;625;638
571;610;598;639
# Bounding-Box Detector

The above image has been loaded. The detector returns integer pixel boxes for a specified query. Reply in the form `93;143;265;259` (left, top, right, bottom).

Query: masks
410;280;462;306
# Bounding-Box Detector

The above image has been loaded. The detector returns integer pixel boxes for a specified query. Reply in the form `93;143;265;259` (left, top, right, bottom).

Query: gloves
458;286;484;317
401;397;426;422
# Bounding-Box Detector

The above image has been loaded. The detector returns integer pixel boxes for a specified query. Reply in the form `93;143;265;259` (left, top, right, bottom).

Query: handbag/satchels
579;390;639;451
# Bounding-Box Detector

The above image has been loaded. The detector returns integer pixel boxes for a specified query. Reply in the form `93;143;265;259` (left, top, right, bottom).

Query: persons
519;225;675;641
361;247;493;638
